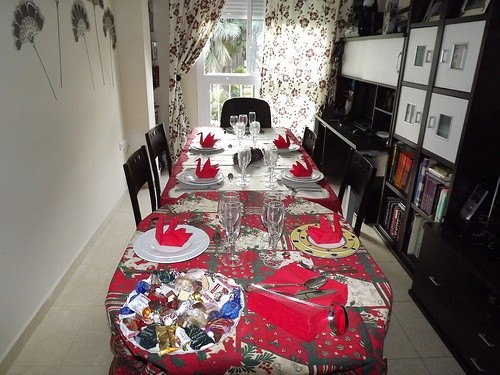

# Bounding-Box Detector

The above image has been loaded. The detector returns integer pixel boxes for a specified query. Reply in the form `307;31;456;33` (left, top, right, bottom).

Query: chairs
123;145;156;227
221;98;271;128
145;124;172;209
302;127;319;161
337;146;377;238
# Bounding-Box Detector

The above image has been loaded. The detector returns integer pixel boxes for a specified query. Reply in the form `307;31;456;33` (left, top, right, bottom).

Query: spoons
297;262;355;272
284;183;322;189
247;275;328;291
212;233;221;258
149;216;204;226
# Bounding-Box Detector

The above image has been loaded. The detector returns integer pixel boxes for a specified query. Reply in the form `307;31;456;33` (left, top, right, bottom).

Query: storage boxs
246;264;348;343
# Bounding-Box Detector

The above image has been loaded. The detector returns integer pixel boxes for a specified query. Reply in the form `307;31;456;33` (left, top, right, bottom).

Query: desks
316;113;391;225
105;192;393;375
160;128;343;213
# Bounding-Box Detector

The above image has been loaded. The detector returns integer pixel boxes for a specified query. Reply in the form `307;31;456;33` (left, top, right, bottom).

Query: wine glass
260;191;285;253
231;111;261;149
235;143;252;187
219;189;245;267
263;201;285;267
258;199;282;261
260;142;280;188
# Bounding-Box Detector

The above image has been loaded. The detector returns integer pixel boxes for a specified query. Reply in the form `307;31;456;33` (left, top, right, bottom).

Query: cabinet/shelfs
340;32;406;148
408;222;500;375
374;0;500;281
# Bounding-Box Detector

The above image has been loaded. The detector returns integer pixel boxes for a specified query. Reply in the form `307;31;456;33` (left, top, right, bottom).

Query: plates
131;125;361;263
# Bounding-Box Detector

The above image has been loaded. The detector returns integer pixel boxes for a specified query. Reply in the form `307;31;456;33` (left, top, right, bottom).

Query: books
383;141;452;259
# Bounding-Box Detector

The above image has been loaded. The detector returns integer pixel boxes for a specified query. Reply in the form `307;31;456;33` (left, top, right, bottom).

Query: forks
307;268;359;277
289;186;323;194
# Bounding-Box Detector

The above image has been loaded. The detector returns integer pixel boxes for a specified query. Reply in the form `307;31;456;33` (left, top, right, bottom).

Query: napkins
289;156;313;177
151;214;193;247
306;213;344;244
196;157;220;178
274;134;291;148
197;132;218;147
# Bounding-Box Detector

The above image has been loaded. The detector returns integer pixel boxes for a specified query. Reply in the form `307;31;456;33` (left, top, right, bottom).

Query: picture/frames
458;0;490;17
